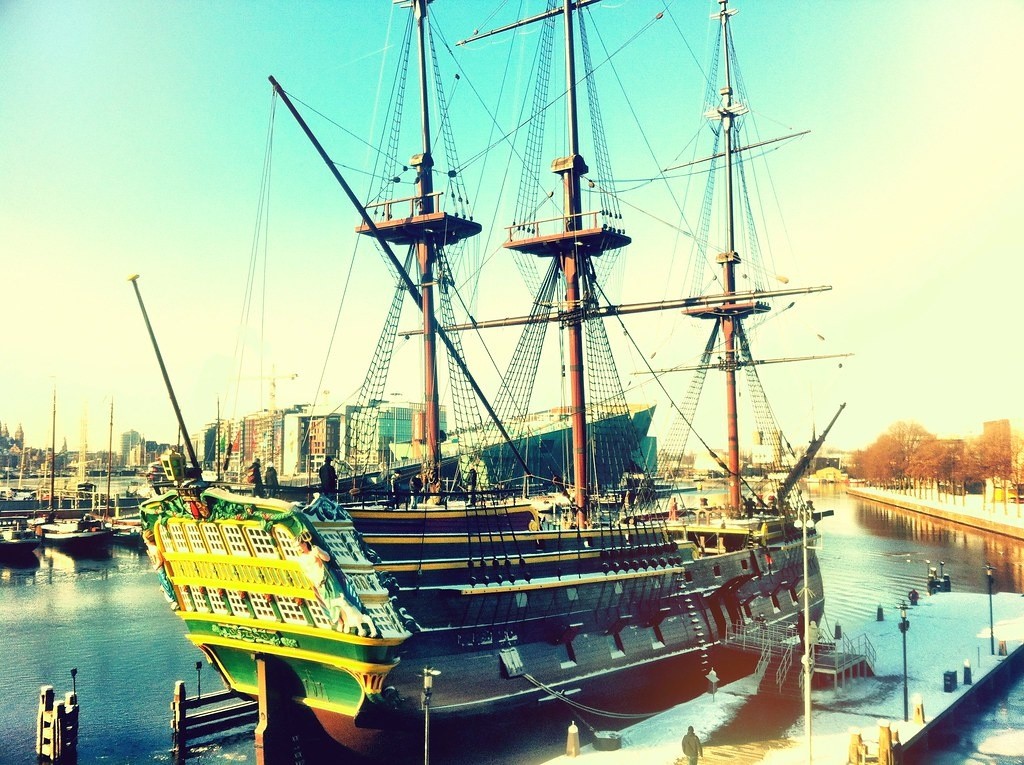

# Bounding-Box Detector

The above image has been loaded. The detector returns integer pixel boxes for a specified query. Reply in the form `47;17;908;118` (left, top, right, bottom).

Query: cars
948;485;966;496
986;484;1019;503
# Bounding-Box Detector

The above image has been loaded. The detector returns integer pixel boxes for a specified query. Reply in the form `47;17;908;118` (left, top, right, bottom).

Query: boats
0;516;41;553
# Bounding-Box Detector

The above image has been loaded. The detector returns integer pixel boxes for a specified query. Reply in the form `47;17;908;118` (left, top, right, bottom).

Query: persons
681;726;703;765
568;522;577;530
464;463;477;507
743;498;756;519
720;520;727;530
408;472;426;509
264;467;279;498
318;456;337;501
769;506;779;516
388;468;404;509
246;459;265;498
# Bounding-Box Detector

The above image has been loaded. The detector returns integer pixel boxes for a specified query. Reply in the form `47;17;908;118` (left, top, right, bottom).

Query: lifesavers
908;591;919;601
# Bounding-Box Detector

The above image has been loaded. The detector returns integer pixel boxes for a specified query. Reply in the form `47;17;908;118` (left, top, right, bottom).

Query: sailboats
27;389;120;546
88;397;143;544
129;0;824;765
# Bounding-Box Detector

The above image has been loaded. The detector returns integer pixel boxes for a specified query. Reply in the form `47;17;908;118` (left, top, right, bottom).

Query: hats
394;468;402;473
324;456;333;462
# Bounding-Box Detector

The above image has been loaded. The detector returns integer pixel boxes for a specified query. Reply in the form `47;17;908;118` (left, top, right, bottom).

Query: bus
828;474;848;481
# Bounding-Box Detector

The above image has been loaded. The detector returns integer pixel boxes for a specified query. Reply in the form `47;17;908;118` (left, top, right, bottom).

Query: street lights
415;665;441;765
894;600;913;722
794;504;814;764
981;563;997;656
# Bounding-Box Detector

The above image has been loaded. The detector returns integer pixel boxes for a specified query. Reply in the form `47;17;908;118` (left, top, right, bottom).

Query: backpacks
248;466;258;482
